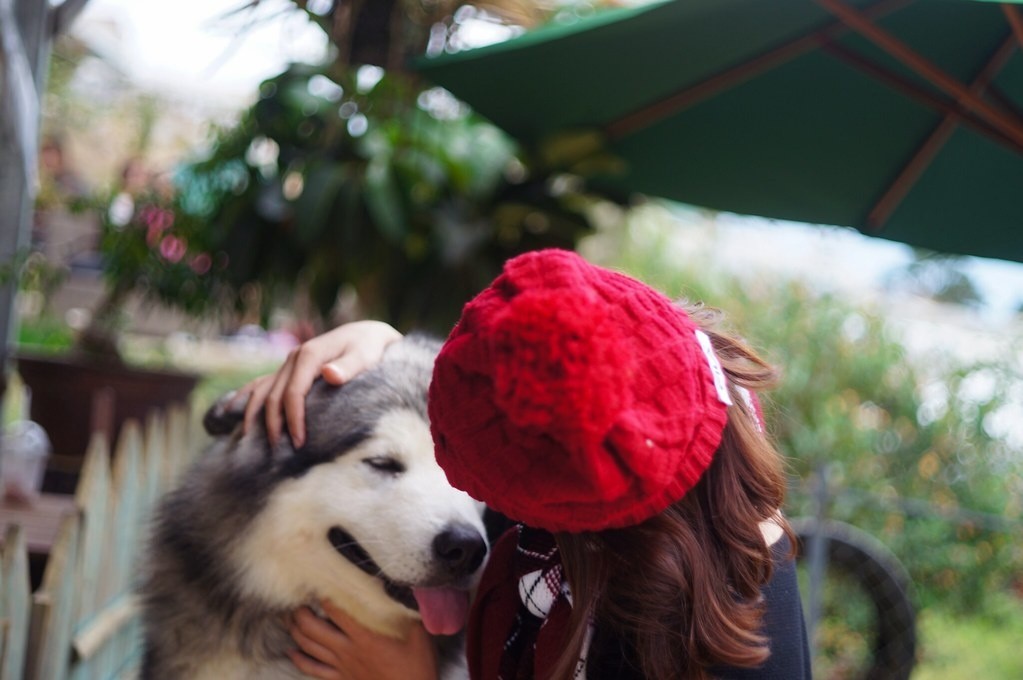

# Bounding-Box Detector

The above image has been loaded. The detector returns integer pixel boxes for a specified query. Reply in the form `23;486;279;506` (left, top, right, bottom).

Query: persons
213;248;814;679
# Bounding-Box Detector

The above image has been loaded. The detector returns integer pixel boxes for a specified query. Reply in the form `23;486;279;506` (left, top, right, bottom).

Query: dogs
135;337;490;680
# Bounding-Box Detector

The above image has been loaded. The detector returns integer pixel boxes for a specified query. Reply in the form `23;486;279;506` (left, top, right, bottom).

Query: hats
428;248;726;530
